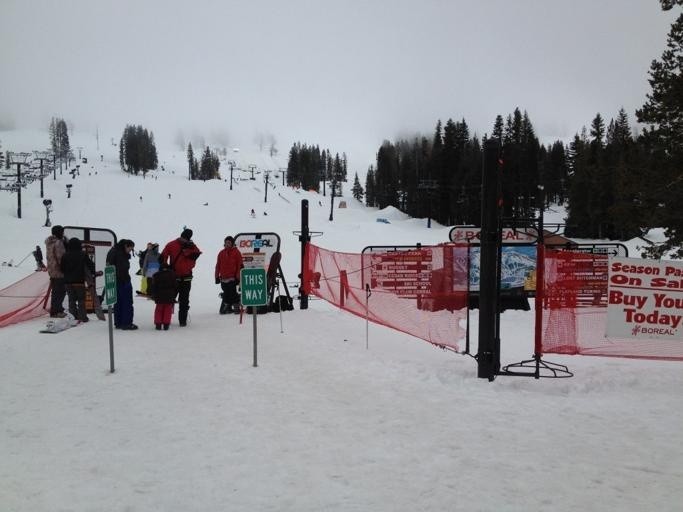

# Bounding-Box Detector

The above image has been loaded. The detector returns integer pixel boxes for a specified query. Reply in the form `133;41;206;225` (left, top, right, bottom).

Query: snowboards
85;269;106;320
262;252;281;313
40;313;81;333
136;290;150;295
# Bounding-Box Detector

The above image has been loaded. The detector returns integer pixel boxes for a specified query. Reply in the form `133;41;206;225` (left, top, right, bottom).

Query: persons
214;235;245;314
106;239;138;330
45;225;68;318
136;228;201;331
60;237;95;323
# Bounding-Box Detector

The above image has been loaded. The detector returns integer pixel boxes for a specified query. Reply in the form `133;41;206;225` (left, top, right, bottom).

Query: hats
181;229;192;239
